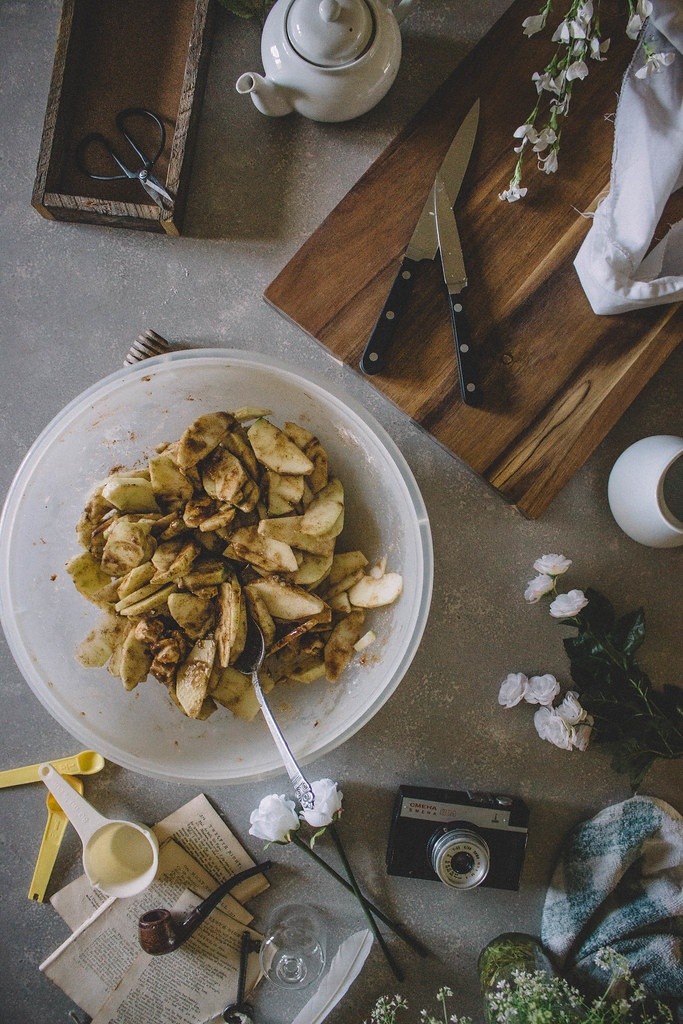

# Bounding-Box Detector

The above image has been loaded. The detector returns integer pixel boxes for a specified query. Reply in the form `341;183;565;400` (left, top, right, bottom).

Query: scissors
74;107;174;211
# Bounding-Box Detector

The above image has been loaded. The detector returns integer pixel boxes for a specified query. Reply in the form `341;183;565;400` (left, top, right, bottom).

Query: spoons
27;775;83;902
234;621;316;811
38;763;159;898
0;751;105;788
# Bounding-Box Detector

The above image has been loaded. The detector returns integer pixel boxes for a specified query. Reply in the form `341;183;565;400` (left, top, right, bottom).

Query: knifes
433;172;485;406
359;99;480;376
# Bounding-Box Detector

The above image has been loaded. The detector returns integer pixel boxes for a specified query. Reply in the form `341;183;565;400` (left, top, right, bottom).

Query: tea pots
235;0;411;123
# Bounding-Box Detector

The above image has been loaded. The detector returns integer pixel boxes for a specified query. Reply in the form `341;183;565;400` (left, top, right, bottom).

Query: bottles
607;435;683;548
259;904;328;991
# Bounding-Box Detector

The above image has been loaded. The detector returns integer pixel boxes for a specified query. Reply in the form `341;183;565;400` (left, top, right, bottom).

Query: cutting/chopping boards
259;0;682;523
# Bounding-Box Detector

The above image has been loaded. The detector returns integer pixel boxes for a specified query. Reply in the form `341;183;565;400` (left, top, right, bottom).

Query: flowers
249;778;432;985
364;946;677;1024
495;553;683;794
497;0;675;204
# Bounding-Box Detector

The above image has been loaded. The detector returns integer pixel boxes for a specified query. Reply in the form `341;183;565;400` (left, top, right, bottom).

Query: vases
477;931;558;1024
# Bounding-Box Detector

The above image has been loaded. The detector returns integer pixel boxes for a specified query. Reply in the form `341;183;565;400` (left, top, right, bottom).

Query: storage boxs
30;0;211;238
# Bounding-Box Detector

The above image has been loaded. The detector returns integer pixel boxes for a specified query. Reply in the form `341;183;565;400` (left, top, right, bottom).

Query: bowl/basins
1;346;434;794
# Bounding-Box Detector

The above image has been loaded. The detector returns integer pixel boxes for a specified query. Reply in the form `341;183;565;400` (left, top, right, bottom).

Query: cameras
385;784;529;892
